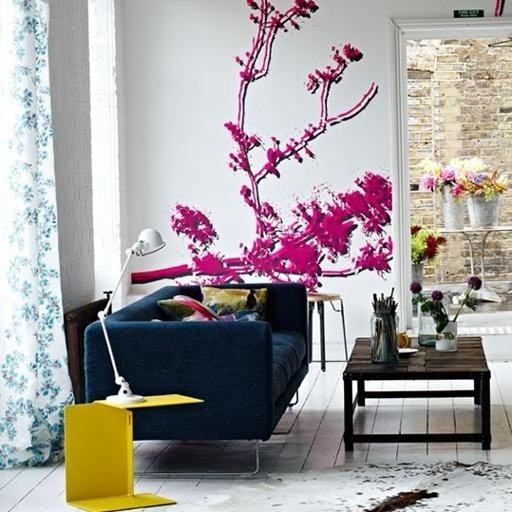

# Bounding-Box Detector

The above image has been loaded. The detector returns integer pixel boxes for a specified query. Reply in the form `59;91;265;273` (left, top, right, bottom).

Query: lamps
97;227;165;404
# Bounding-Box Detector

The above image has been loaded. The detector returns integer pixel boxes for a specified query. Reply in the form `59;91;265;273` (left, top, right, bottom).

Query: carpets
185;460;512;512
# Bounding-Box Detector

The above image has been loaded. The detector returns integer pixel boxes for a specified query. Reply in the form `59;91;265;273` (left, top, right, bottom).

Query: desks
435;226;512;304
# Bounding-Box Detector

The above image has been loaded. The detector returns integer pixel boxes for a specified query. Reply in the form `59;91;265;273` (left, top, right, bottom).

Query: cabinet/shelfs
63;392;204;512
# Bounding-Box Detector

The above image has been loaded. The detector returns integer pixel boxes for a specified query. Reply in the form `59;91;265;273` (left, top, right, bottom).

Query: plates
400;348;418;354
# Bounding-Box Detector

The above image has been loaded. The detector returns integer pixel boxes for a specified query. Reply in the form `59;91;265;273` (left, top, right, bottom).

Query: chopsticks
371;286;398;313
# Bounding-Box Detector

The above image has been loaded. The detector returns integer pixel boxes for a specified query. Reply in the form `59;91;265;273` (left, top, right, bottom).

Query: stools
308;293;349;371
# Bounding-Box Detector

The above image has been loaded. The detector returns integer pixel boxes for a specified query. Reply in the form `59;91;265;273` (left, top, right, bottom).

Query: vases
442;186;465;230
411;263;425;290
418;290;449;347
466;195;500;228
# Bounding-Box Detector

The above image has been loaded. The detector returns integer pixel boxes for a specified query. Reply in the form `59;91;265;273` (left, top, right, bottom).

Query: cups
400;331;409;349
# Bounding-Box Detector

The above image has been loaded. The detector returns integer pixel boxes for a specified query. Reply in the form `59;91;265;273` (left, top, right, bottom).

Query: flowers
418;155;487;193
452;165;512;202
410;225;447;265
409;276;482;335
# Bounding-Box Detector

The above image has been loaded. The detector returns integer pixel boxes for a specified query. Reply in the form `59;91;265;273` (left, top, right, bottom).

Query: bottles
418;314;435;346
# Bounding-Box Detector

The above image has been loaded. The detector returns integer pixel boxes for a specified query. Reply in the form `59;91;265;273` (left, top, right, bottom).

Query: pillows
158;287;268;323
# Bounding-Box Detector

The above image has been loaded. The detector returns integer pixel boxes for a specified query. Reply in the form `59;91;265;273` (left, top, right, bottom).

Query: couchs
84;283;308;477
